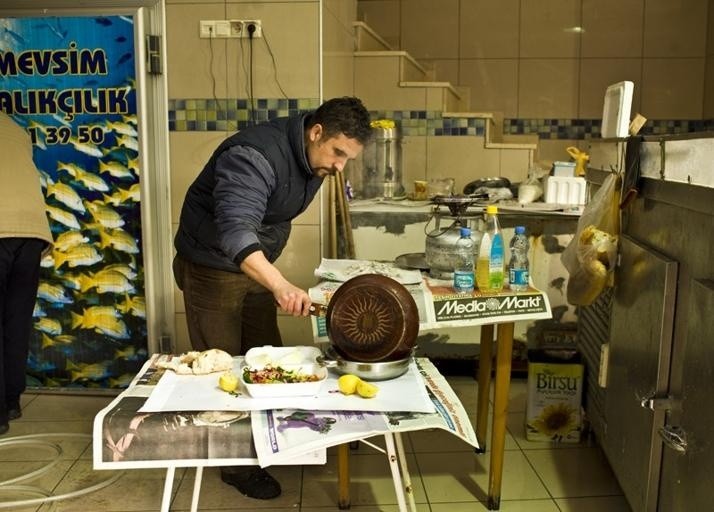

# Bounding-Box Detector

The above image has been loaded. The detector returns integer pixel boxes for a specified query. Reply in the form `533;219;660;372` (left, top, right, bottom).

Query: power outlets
243;20;261;38
230;20;243;38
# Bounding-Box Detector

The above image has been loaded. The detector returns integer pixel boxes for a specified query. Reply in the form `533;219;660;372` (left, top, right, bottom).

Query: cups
414;179;430;201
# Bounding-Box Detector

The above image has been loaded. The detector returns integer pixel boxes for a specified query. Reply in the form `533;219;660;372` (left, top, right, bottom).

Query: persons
172;97;373;356
0;108;55;436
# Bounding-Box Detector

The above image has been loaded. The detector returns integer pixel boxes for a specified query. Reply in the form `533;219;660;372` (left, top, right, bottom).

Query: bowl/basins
317;342;419;383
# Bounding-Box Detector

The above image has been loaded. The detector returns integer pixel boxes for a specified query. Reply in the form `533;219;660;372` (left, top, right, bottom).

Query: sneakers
222;467;281;499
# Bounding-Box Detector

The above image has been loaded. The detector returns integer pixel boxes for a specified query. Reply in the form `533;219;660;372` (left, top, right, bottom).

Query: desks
91;355;481;511
308;261;554;509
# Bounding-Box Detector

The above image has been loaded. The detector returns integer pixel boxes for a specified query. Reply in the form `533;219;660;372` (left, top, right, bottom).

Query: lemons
338;375;361;395
357;381;378;397
219;373;238;392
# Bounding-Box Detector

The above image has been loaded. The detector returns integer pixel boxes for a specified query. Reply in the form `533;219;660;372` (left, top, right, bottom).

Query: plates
240;361;330;398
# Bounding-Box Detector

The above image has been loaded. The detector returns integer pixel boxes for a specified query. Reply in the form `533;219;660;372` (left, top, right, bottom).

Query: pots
275;273;421;362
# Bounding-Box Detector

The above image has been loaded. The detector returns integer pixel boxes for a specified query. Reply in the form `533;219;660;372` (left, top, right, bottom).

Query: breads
565;225;615;306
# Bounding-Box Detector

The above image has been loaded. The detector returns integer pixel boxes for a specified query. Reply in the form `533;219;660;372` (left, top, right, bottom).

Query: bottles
453;205;531;294
